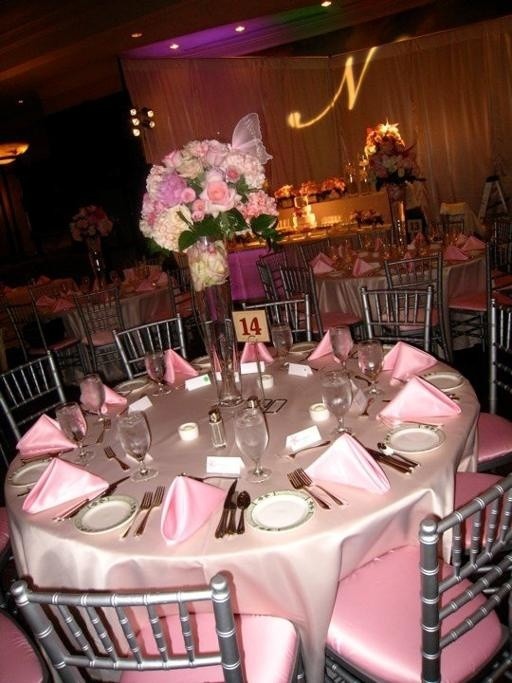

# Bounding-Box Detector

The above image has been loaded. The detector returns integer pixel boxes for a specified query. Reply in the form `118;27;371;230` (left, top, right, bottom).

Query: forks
287;467;343;510
96;418;112;444
120;485;166;539
376;442;421;467
102;446;132;472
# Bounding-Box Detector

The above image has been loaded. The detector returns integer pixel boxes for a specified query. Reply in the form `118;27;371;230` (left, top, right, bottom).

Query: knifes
215;479;239;539
367;448;413;475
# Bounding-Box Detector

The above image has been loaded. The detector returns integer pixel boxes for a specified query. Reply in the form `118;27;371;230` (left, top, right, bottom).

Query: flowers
139;140;278;252
274;183;296;198
361;124;423;190
321;178;345;193
299;180;320;195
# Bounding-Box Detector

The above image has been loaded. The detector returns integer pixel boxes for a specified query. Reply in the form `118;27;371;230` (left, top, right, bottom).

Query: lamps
129;106;155;137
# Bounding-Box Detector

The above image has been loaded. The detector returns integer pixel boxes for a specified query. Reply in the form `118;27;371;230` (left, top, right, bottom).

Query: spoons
236;490;250;534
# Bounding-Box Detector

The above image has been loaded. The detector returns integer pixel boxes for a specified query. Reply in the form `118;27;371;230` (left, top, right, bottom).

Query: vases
306;195;317;203
328;189;340;198
283;199;292;208
386;185;406;249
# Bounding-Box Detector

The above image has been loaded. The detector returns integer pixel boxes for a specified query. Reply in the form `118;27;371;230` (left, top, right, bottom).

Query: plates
73;494;139;535
115;378;147;394
289;341;318;354
384;424;446;454
421;370;465;393
244;489;316;533
190;355;211;368
7;457;53;489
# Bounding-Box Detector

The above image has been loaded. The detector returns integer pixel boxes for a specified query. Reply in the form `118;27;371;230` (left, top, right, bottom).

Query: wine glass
54;374;110;462
117;410;159;484
357;339;384;399
271;320;294;374
233;407;274;484
144;347;172;398
59;267;151;300
320;368;355;439
329;324;353;372
330;226;463;271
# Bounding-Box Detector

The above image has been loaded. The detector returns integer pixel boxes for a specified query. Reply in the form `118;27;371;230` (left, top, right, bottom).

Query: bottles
208;409;227;449
247;396;263;413
